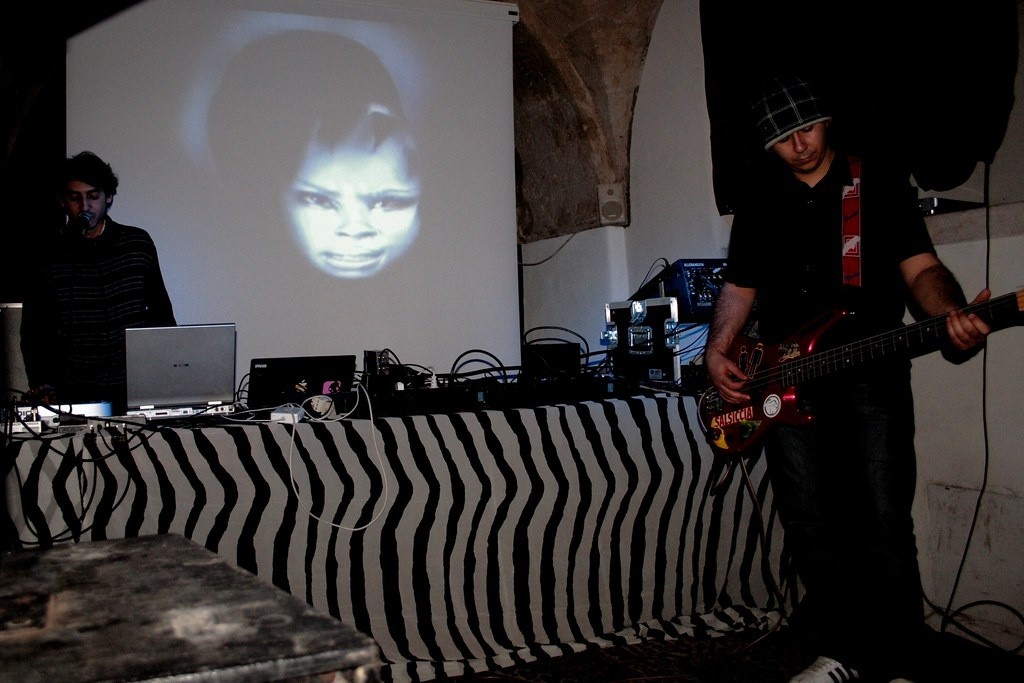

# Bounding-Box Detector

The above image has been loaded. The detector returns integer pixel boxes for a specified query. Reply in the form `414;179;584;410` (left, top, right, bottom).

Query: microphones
77;212;92;235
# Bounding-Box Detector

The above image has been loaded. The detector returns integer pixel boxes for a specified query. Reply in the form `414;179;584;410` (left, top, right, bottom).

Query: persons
704;76;992;683
281;90;423;281
24;151;178;416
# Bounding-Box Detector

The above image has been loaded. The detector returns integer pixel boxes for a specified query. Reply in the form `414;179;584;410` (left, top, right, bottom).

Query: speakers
597;183;628;226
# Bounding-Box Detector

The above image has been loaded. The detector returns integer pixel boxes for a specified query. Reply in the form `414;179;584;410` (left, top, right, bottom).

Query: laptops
126;323;238;416
247;355;357;414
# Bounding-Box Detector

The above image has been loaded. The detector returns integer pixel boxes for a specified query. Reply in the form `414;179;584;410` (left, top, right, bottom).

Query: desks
0;532;382;682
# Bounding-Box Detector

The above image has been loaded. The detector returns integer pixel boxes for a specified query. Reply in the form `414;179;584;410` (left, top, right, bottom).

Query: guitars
698;286;1024;455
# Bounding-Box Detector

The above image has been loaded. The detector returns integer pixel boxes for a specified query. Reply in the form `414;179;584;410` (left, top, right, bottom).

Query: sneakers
790;656;858;683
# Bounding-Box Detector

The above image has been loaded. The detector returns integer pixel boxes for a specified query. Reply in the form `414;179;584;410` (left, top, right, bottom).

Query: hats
747;76;834;150
